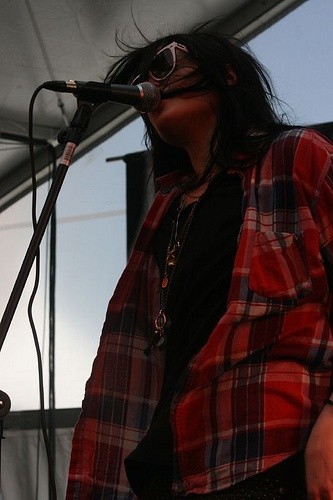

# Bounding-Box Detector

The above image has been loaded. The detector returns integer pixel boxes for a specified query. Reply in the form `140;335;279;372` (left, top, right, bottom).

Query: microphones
42;80;162;112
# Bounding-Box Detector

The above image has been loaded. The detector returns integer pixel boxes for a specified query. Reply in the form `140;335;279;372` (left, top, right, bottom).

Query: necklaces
148;178;209;348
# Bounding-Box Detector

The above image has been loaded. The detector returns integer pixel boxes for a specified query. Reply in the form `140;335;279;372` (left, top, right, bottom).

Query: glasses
130;40;191;87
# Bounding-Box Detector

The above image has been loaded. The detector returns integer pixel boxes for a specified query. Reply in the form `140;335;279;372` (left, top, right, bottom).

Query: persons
64;28;333;500
